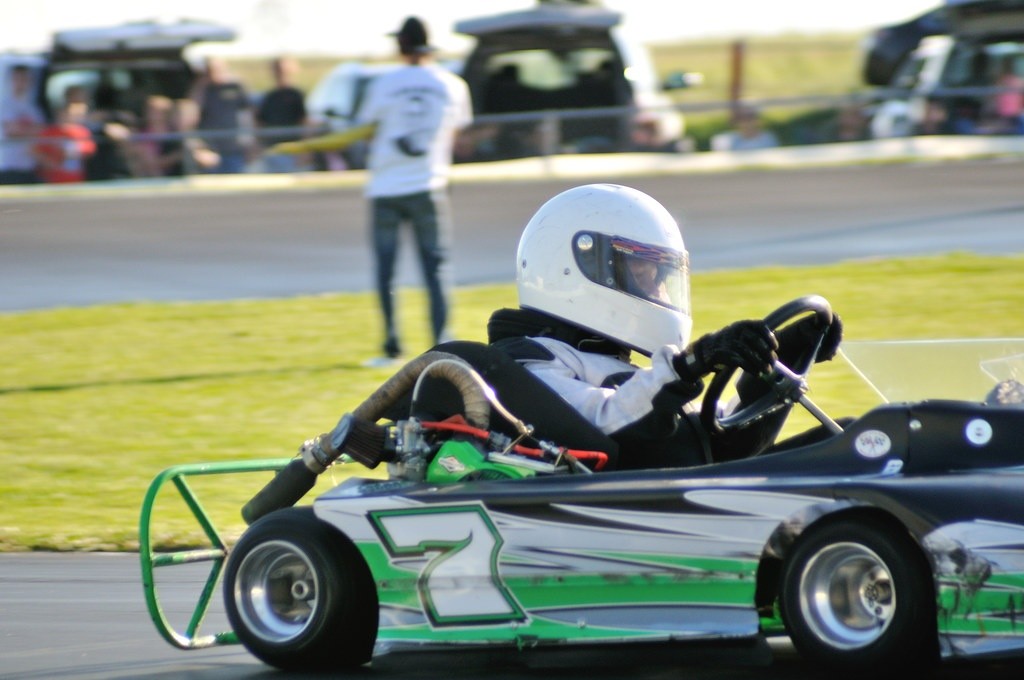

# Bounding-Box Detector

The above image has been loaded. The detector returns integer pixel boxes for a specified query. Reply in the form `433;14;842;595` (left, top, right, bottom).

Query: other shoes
364;352;402;371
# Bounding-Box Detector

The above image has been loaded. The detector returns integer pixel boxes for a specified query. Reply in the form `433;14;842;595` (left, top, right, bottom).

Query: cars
451;0;684;162
33;18;237;134
863;0;1023;141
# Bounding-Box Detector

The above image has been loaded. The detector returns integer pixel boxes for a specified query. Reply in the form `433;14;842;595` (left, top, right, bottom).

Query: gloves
697;320;778;379
769;313;841;370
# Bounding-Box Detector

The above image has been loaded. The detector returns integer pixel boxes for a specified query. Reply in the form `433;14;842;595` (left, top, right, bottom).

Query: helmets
515;184;695;358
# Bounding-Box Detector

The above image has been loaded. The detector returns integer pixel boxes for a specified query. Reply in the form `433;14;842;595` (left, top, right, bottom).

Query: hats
629;109;684;143
735;101;761;125
397;17;433;55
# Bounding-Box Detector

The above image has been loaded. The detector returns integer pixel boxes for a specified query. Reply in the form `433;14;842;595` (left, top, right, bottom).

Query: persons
182;60;258;178
610;30;1021;147
484;179;859;464
355;19;475;367
253;57;308;171
5;62;179;178
